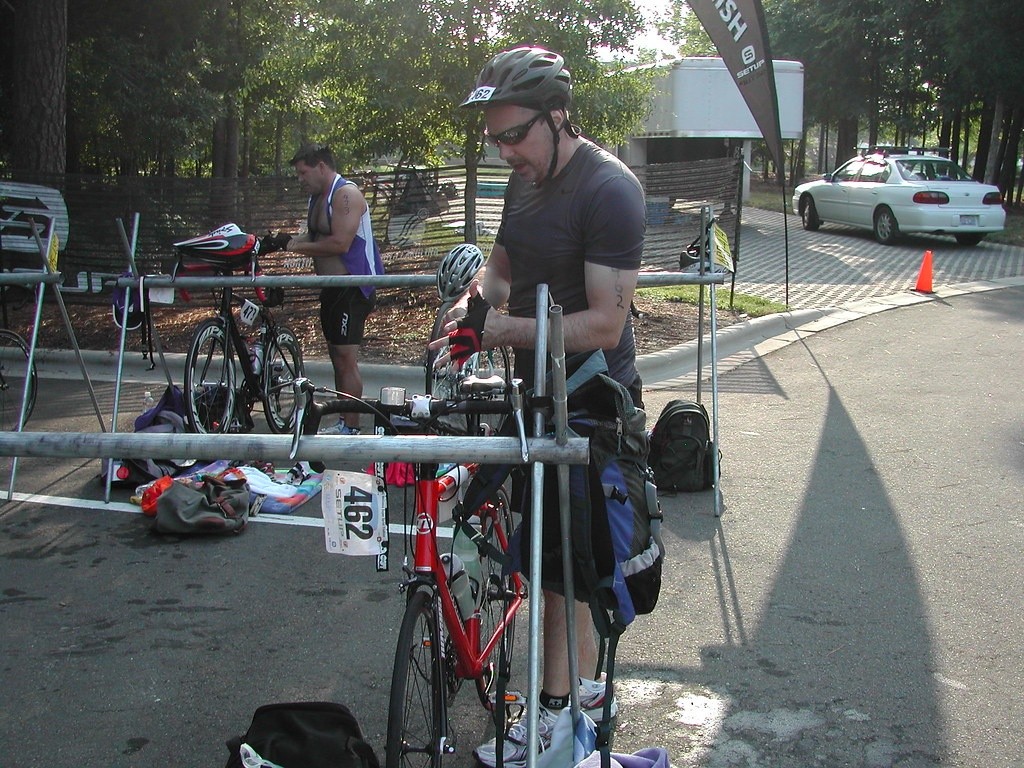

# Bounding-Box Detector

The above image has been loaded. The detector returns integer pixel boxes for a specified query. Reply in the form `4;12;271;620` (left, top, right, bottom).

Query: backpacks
647;399;722;494
225;702;379;768
452;349;666;638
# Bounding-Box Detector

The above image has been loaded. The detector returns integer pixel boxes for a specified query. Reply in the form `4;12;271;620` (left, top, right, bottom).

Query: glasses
483;111;542;146
239;743;284;768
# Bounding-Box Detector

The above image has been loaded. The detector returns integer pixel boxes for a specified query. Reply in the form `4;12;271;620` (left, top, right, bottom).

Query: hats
112;272;143;331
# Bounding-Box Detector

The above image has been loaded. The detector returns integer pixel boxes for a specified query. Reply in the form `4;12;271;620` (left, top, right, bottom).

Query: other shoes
338;425;360;435
316;420;344;435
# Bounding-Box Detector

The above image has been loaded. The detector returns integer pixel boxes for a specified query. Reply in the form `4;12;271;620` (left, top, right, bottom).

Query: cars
792;153;1007;244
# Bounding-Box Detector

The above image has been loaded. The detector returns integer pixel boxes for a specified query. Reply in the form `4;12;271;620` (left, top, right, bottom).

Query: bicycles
172;237;305;439
268;375;552;768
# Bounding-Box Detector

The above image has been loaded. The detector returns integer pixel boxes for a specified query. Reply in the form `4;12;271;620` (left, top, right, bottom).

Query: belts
139;275;155;370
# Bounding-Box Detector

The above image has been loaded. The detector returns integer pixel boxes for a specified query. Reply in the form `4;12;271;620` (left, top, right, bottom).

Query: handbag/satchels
153;474;251;536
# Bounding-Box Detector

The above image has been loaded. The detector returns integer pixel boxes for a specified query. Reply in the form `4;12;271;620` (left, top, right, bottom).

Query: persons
258;143;384;435
429;47;648;768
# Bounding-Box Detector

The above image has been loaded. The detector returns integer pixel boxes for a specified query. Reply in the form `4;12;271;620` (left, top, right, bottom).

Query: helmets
679;245;730;275
437;243;485;302
458;45;571;106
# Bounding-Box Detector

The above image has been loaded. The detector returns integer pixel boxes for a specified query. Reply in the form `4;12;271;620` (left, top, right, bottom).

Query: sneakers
471;701;560;768
572;677;618;722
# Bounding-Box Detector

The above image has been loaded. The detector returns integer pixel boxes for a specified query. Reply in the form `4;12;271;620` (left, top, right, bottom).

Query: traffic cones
912;250;935;294
